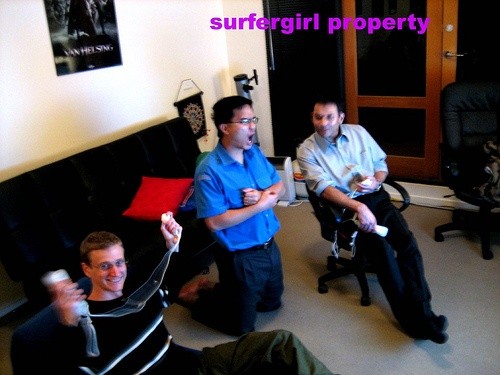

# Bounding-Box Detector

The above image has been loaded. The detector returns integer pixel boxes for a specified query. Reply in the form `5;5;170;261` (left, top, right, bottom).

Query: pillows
121;175;194;219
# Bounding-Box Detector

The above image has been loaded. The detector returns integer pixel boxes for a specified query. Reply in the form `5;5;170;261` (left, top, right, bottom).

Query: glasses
228;118;258;125
89;259;127;270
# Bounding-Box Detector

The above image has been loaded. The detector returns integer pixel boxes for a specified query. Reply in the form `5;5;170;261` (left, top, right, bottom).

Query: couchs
0;118;214;318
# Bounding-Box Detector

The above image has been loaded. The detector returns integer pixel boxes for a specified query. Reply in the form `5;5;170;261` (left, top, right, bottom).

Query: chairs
297;133;411;306
433;80;500;259
10;276;202;375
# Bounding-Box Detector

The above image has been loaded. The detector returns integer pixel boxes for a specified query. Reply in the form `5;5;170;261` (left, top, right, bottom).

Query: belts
229;237;275;254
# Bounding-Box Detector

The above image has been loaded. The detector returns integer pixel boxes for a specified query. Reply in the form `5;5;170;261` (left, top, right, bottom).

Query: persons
51;211;335;375
179;96;286;335
296;97;449;344
